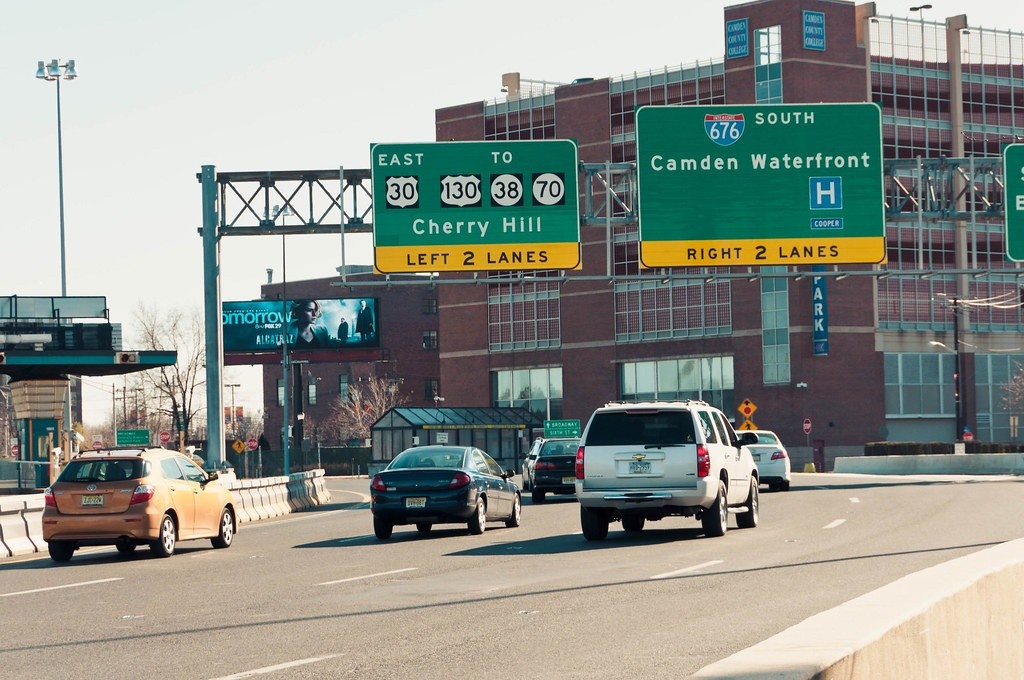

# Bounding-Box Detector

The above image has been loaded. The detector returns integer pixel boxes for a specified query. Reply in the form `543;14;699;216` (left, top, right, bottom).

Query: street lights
224;384;241;435
910;4;937;329
34;58;80;299
262;204;297;476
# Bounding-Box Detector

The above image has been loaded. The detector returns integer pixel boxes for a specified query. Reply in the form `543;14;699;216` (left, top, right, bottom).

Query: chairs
417;457;435;466
102;464;127;482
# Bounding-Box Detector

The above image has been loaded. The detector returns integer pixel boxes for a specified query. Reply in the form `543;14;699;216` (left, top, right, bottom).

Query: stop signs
248;438;258;450
11;445;18;457
93;442;102;450
160;432;170;442
802;418;812;434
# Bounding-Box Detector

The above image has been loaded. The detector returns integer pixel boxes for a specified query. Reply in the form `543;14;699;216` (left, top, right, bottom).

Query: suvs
41;447;238;563
521;436;581;503
574;399;759;543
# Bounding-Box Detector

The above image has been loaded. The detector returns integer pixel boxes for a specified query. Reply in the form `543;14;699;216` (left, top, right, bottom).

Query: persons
356;300;373;341
288;299;329;345
338;318;348;342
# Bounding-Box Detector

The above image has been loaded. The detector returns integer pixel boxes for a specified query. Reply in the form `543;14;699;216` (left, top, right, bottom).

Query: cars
370;444;522;542
733;430;791;492
